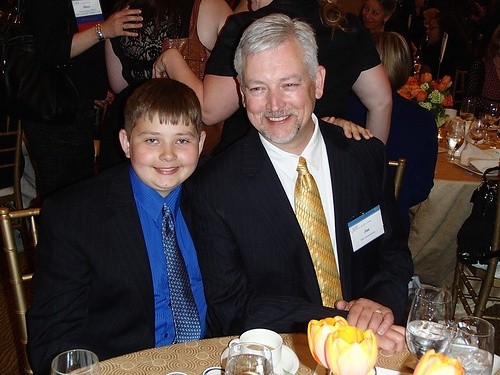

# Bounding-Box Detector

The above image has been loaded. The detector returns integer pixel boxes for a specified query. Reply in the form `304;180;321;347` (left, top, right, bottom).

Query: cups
444;315;495;375
50;349;100;375
229;329;283;367
405;287;453;360
467;116;487;148
460;96;476;122
445;109;457;118
436;119;450;154
224;342;274;375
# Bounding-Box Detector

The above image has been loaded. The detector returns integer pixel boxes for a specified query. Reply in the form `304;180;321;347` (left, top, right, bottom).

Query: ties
161;201;202;345
294;156;344;309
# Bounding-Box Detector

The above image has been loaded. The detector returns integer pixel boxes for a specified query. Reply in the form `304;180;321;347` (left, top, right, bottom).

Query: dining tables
67;320;500;375
407;112;500;288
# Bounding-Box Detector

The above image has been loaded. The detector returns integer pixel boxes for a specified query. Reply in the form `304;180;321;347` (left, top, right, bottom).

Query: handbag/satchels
455;166;500;263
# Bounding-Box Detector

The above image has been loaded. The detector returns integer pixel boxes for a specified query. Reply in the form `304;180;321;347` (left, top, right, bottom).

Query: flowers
398;73;454;127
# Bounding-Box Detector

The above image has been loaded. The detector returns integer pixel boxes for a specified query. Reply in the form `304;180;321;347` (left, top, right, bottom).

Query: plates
221;345;300;375
454;161;499;177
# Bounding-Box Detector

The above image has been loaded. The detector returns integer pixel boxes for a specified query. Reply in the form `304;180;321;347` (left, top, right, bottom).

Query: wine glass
444;119;467;163
482;101;500;150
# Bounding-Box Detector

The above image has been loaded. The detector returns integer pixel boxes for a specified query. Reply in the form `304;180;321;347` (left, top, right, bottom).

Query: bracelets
95;24;105;41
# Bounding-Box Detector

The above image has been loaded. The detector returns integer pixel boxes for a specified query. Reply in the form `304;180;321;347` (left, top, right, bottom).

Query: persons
104;0;392;146
191;13;416;355
401;0;500;118
344;31;438;246
26;78;374;375
357;0;414;77
14;0;144;206
10;128;36;255
151;0;236;158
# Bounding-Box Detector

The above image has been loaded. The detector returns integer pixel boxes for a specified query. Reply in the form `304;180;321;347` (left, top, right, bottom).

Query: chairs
0;114;32;273
0;204;41;375
450;198;500;321
